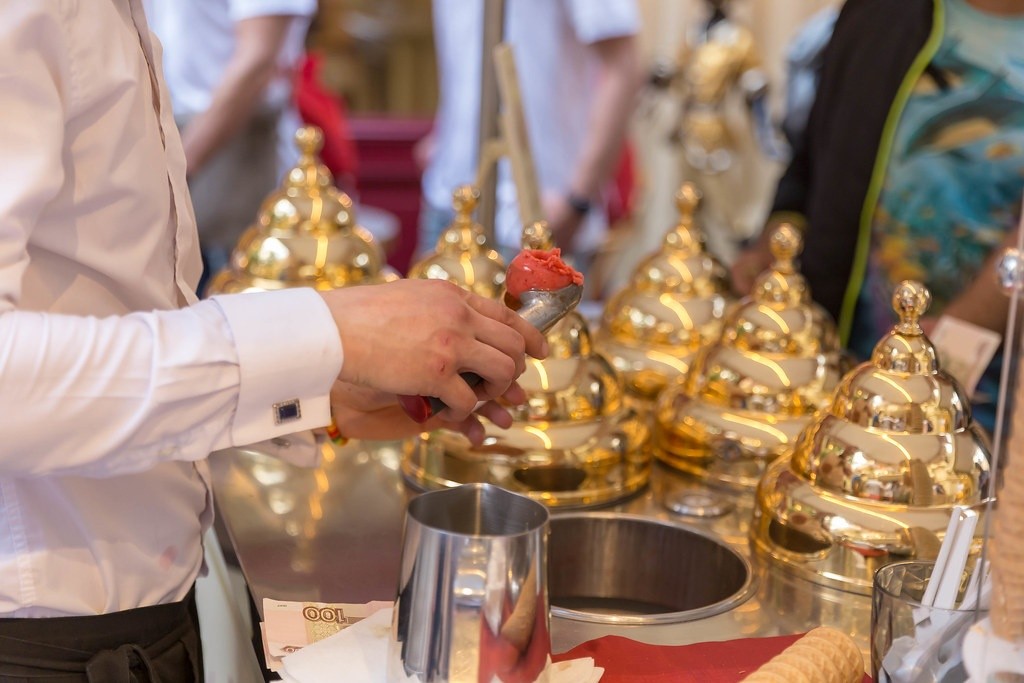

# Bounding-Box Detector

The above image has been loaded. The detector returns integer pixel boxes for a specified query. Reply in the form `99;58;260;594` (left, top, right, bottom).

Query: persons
733;0;1024;438
141;0;638;300
1;0;551;682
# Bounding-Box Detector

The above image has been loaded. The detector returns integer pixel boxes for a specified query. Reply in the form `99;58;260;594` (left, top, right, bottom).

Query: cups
387;483;552;683
871;561;990;683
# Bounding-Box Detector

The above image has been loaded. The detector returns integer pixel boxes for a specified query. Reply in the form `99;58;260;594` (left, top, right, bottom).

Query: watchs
567;192;590;216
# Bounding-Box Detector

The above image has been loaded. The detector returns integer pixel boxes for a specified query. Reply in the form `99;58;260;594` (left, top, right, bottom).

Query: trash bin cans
327;114;435;278
